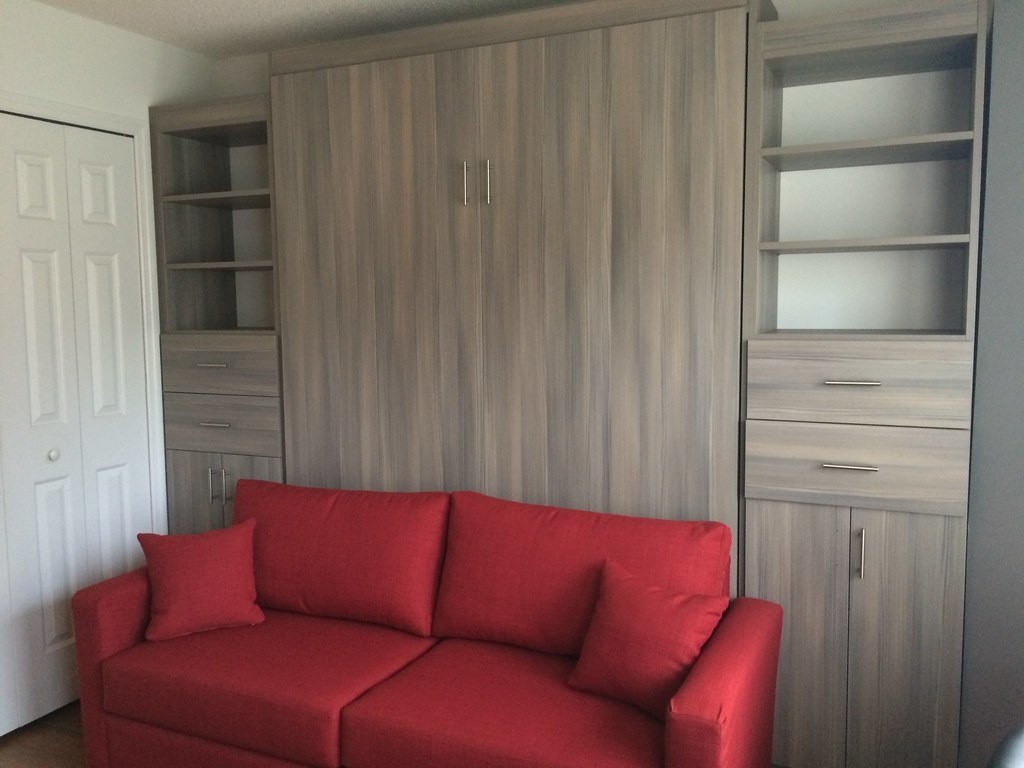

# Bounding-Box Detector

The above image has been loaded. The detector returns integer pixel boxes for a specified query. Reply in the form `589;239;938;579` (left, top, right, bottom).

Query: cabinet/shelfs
148;96;283;536
747;1;992;766
271;1;768;596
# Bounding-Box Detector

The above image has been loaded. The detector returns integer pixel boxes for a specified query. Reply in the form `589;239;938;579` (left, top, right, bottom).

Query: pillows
568;556;729;723
136;518;266;642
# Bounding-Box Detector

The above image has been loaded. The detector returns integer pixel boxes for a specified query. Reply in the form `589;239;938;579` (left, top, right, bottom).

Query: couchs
72;478;784;768
